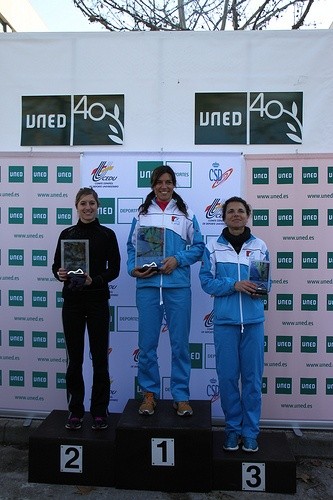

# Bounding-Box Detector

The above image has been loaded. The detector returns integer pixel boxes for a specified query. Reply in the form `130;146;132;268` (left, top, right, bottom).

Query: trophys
135;226;165;270
61;240;89;277
247;259;271;295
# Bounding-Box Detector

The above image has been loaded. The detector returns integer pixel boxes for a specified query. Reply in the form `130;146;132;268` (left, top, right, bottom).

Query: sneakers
91;417;108;429
172;401;193;416
242;440;258;452
138;393;156;415
223;432;241;450
64;412;83;429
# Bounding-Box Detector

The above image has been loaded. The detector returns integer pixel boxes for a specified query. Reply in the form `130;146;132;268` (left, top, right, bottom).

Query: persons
51;187;121;430
126;166;205;417
199;196;272;452
